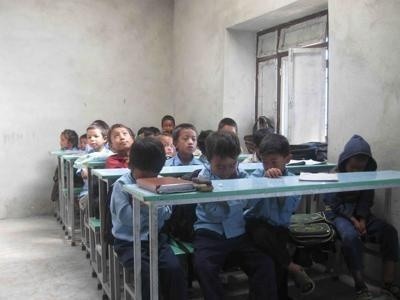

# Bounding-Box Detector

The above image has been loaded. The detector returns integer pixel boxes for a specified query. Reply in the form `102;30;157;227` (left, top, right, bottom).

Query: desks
52;149;339;299
122;170;399;300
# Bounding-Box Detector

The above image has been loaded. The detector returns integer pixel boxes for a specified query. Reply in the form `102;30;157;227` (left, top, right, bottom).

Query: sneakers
381;280;400;299
354;282;372;299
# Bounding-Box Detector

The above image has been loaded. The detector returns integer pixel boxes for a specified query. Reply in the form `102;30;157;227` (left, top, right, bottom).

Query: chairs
319;166;388;298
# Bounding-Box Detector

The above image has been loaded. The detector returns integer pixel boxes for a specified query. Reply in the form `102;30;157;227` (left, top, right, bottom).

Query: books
135;174;193;193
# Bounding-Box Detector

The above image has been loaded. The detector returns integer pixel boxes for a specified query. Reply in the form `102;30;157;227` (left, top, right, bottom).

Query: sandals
291;265;315;296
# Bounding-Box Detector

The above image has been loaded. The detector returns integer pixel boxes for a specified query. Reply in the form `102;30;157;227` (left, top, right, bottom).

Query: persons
191;130;280;299
51;112;286;244
108;137;190;300
246;134;317;295
325;135;400;297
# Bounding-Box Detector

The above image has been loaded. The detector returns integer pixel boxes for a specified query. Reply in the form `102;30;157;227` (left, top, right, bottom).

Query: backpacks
289;210;336;250
174;169;202;242
289;142;327;162
244;116;274;154
